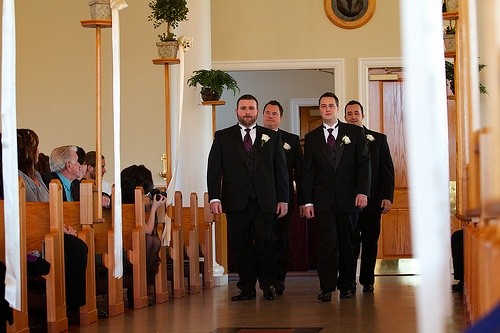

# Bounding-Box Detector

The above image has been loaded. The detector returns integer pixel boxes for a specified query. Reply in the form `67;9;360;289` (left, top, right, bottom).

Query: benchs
0;175;228;333
463;128;500;323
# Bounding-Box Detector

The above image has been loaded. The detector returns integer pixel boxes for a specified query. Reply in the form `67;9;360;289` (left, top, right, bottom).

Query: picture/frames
323;0;376;29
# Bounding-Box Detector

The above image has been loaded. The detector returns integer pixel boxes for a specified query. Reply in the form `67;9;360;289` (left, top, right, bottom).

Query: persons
120;165;167;308
337;100;395;293
262;100;305;296
302;91;371;301
206;94;290;301
451;229;464;291
0;128;111;325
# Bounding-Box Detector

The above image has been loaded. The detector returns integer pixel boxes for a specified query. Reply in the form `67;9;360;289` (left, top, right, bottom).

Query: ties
323;123;338;146
239;126;256;154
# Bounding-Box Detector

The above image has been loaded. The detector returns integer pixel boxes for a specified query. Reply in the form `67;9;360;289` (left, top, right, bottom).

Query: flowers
283;142;292;150
339;133;351;147
261;133;270;147
366;134;375;145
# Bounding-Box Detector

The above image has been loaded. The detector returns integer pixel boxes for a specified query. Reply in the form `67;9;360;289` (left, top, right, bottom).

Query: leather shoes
262;284;274;300
318;291;331;301
363;283;374;292
231;293;256;301
340;289;352;299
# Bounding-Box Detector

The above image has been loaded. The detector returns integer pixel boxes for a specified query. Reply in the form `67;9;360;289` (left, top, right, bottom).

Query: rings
211;211;212;213
364;203;366;204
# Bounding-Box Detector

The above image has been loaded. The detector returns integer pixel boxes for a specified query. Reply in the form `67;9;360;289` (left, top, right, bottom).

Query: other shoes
275;281;286;295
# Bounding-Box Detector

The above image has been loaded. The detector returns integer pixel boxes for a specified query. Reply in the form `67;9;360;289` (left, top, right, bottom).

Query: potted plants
444;26;456;53
147;0;189;58
187;69;240;101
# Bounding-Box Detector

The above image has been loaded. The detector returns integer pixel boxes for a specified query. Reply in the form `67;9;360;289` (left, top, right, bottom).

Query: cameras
149;190;167;202
